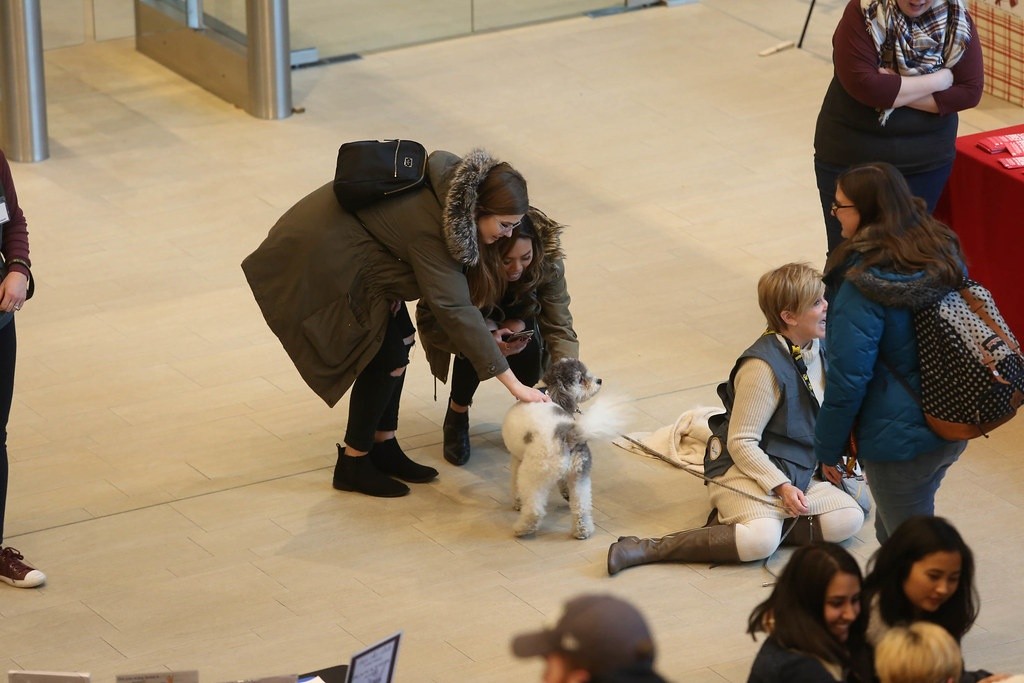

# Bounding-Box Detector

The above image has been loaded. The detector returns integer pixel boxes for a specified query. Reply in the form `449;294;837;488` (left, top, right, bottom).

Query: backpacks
334;139;442;214
875;274;1024;440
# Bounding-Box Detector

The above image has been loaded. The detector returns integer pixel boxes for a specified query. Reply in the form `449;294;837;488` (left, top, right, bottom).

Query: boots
607;524;742;575
703;507;824;546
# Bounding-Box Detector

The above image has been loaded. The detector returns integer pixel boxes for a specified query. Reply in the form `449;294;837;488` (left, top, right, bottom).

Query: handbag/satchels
821;459;871;516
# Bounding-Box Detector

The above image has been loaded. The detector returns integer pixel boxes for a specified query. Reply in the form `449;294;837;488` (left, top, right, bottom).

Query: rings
13;305;20;311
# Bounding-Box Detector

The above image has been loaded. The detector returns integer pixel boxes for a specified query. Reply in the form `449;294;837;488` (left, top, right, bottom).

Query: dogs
502;358;628;540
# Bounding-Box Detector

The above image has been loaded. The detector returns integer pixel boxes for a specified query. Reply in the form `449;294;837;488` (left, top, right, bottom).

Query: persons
0;142;48;597
512;593;667;683
860;516;1021;683
416;208;580;467
873;623;961;683
239;138;527;500
814;0;985;283
746;540;877;683
815;162;968;545
607;265;870;575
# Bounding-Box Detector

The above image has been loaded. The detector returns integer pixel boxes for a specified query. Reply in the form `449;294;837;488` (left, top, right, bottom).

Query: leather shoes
442;398;470;465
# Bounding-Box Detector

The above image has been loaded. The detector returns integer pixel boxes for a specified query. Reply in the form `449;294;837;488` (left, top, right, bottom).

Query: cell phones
507;329;534;342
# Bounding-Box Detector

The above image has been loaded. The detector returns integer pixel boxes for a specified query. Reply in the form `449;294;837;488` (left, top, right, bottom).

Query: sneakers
0;547;46;588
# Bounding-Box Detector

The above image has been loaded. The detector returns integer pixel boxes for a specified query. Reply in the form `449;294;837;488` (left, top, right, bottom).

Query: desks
967;0;1024;108
933;123;1024;356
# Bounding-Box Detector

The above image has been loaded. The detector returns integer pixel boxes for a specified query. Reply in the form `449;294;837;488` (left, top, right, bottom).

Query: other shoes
333;442;410;498
372;436;439;483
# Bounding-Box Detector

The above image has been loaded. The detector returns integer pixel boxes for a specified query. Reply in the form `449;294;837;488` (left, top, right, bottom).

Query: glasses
831;201;856;212
492;215;522;234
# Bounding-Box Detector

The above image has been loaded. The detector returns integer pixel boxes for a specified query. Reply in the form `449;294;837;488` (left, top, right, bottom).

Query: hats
514;596;654;676
